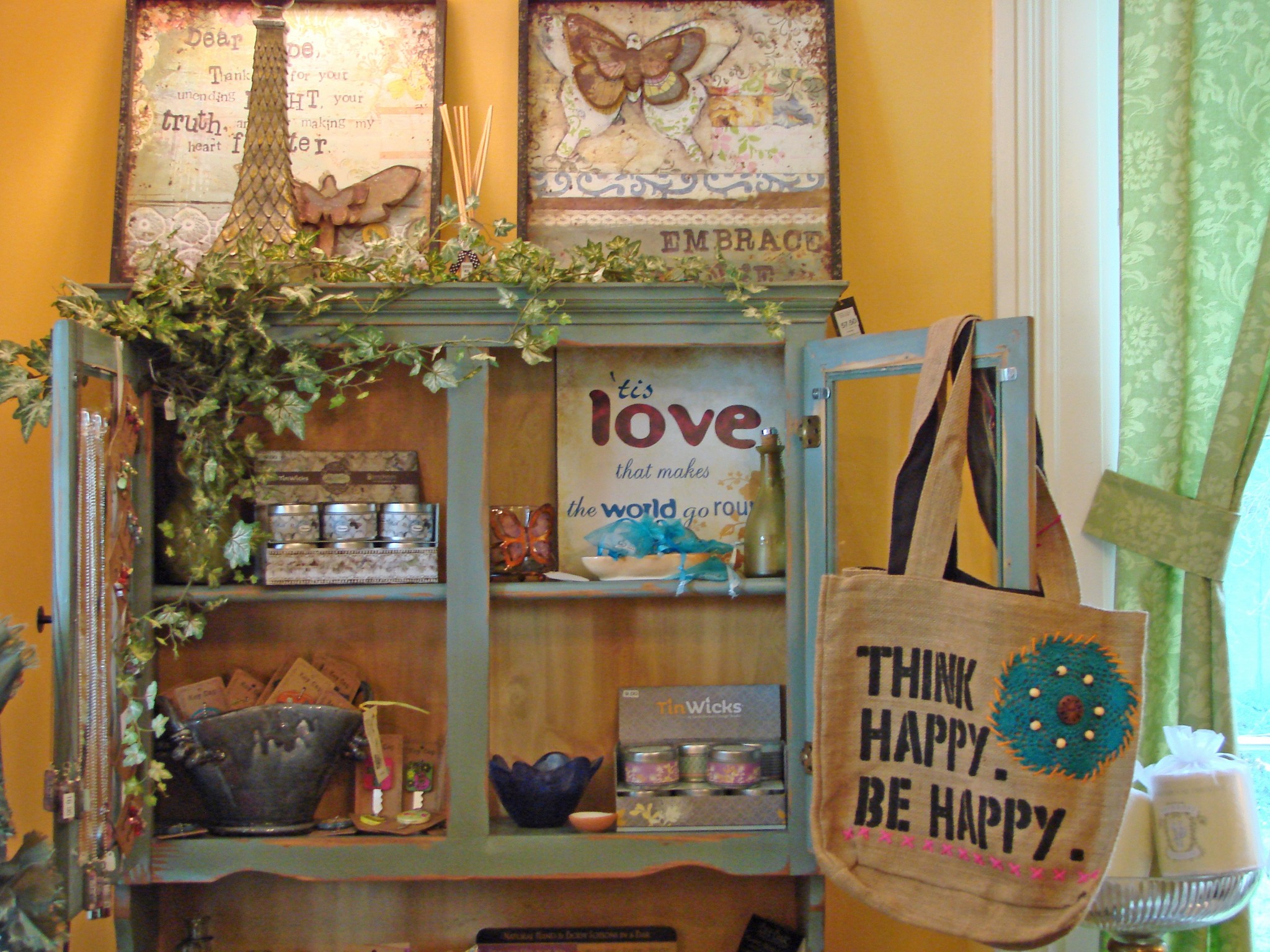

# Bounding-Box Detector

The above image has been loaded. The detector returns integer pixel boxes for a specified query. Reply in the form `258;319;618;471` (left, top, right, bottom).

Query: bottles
174;915;214;952
744;427;788;577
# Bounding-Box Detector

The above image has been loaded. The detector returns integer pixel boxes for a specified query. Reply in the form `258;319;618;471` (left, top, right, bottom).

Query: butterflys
537;11;741;164
492;503;555;570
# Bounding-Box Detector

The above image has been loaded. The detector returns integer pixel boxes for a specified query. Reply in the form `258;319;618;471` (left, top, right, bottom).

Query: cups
489;504;558;581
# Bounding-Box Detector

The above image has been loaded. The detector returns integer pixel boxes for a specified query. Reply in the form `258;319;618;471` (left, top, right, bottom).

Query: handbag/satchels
807;312;1145;949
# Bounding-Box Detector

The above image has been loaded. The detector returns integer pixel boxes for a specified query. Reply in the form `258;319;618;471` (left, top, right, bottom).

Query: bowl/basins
568;812;616;832
172;705;362;833
488;751;606;828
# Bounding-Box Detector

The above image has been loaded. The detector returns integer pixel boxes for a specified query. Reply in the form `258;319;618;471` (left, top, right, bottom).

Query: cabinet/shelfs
49;278;1033;952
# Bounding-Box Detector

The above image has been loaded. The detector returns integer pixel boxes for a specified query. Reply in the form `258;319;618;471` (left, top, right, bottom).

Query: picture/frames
517;0;844;279
107;0;449;281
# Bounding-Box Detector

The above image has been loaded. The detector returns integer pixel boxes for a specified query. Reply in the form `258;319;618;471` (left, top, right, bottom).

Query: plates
582;553;711;581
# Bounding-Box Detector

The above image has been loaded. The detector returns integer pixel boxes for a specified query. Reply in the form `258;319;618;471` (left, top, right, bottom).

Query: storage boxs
253;451;439;586
614;684;787;827
